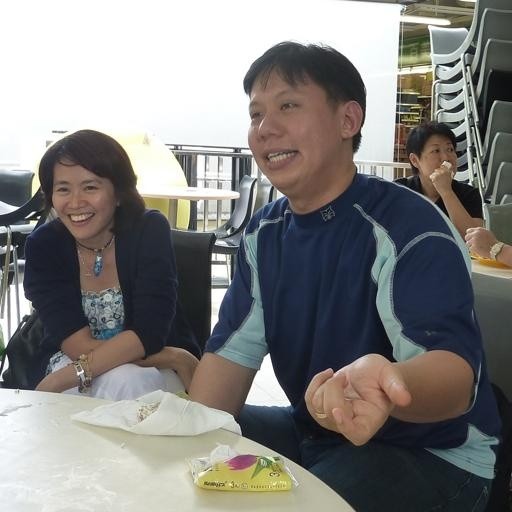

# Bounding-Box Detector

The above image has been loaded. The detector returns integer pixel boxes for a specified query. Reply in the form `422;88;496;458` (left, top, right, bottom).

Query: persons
388;120;485;241
1;128;201;402
463;227;512;268
189;38;508;512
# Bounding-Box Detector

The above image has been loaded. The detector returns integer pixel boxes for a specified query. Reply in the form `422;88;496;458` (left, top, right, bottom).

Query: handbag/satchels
481;379;511;512
6;306;59;391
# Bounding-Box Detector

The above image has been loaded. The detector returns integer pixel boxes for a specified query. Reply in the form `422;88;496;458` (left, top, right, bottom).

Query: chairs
427;0;512;255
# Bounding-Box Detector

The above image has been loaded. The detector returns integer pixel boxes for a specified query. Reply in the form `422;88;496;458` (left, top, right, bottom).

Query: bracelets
72;352;94;394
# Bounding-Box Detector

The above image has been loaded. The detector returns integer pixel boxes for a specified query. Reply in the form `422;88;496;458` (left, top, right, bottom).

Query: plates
470;253;510;269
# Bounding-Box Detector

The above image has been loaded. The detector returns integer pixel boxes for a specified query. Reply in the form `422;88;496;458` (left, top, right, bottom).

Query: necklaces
72;236;115;278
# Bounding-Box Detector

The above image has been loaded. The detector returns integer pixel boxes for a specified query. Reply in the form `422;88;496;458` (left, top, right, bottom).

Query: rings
314;411;327;421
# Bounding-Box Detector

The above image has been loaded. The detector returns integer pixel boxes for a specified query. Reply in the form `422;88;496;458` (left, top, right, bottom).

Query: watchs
490;241;503;261
